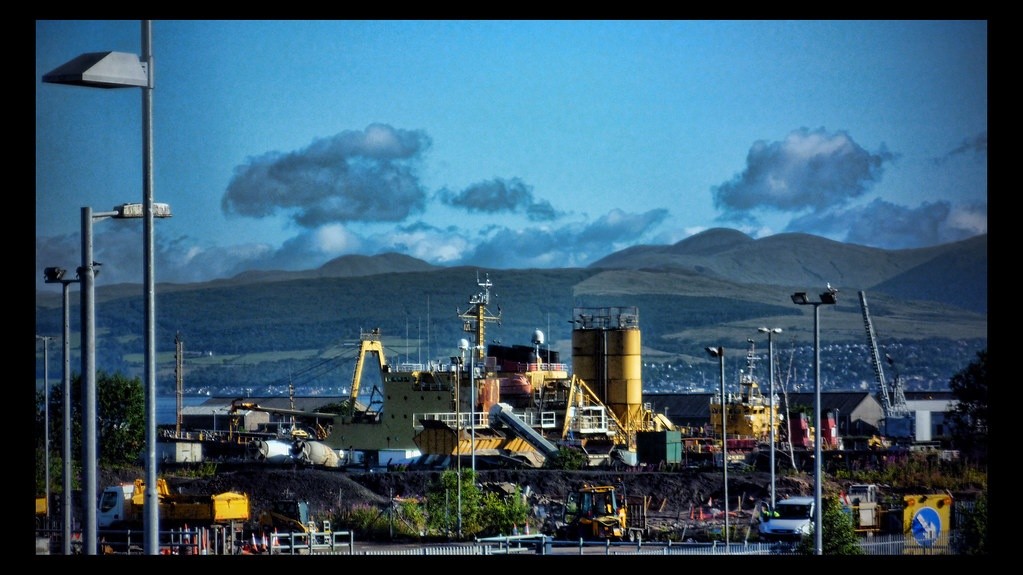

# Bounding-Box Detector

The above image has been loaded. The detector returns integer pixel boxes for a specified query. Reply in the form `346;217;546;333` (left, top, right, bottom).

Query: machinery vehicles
561;479;645;541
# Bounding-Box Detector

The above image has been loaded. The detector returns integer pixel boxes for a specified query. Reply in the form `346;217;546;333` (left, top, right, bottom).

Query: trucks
98;476;251;546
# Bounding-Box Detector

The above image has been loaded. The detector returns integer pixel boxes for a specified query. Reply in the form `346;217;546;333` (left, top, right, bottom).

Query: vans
758;497;818;545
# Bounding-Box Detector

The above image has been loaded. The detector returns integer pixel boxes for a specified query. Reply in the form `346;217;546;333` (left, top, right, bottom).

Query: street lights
704;343;729;546
758;326;783;514
80;203;174;554
789;289;839;558
42;19;159;557
44;263;103;557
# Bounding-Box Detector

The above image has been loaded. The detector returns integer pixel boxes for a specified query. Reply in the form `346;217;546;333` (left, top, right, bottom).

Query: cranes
858;289;911;418
